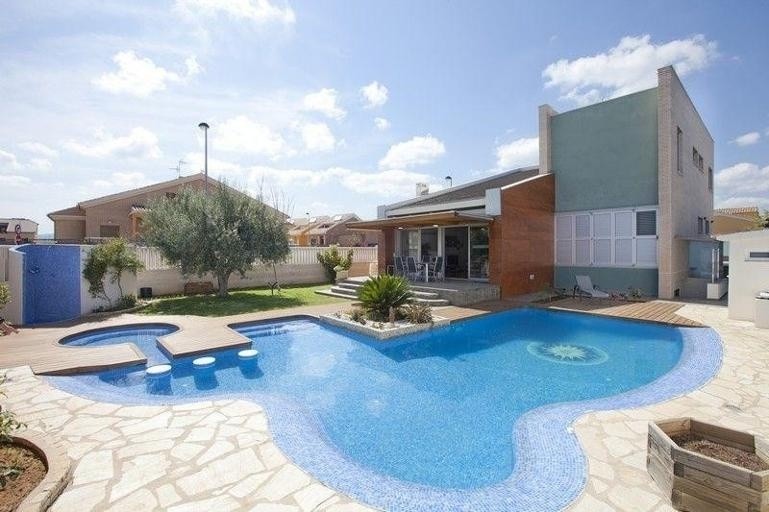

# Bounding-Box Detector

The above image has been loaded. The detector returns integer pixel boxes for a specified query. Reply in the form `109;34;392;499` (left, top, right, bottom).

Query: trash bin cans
752;296;769;329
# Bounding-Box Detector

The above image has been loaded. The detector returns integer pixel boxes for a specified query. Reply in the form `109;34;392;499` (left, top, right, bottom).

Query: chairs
386;253;444;284
573;274;609;302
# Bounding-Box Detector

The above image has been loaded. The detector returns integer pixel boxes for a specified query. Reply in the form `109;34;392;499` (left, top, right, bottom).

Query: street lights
305;212;311;246
197;121;211;194
443;174;453;188
